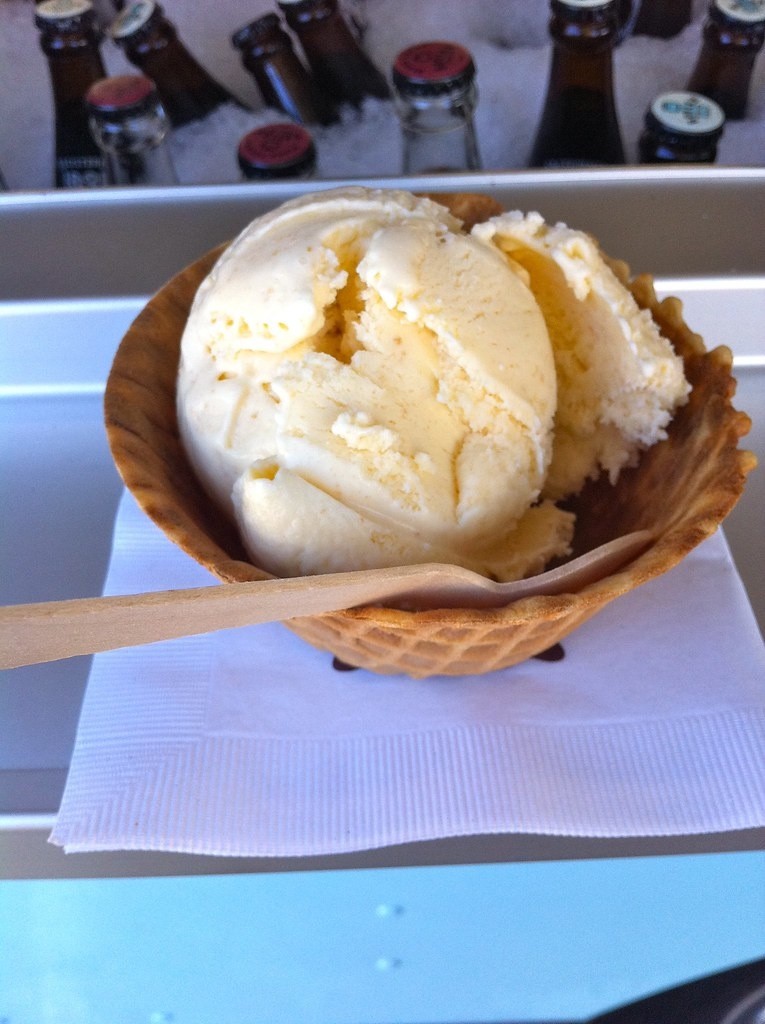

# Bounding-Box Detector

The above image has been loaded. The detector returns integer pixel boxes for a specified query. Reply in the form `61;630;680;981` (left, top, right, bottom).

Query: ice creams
172;179;692;579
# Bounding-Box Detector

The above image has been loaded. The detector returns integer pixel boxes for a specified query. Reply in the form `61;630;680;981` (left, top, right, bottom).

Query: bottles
29;0;765;193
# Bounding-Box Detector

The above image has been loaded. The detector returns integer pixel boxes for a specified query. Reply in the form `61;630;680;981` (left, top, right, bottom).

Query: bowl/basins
103;240;757;678
